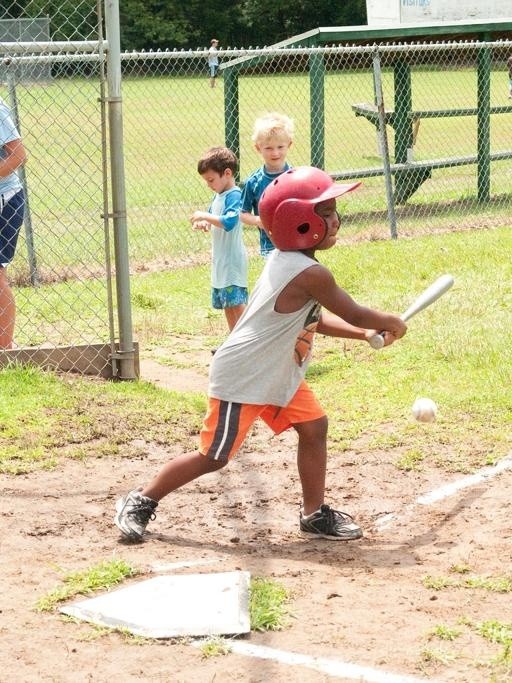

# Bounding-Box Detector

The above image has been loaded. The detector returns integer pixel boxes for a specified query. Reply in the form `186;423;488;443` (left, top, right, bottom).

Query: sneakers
114;487;158;542
300;504;363;541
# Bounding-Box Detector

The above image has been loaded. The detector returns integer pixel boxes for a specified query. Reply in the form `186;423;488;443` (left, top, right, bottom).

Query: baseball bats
371;274;454;348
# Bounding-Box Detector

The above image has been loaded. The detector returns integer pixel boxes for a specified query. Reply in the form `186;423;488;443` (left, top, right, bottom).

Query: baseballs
413;397;436;422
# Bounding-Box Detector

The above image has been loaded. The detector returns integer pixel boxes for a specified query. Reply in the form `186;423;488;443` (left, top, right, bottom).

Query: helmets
258;166;363;251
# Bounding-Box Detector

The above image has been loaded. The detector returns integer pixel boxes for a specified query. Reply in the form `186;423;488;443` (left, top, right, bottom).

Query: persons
0;95;29;350
189;146;249;355
239;112;295;256
207;38;220;88
114;163;408;544
